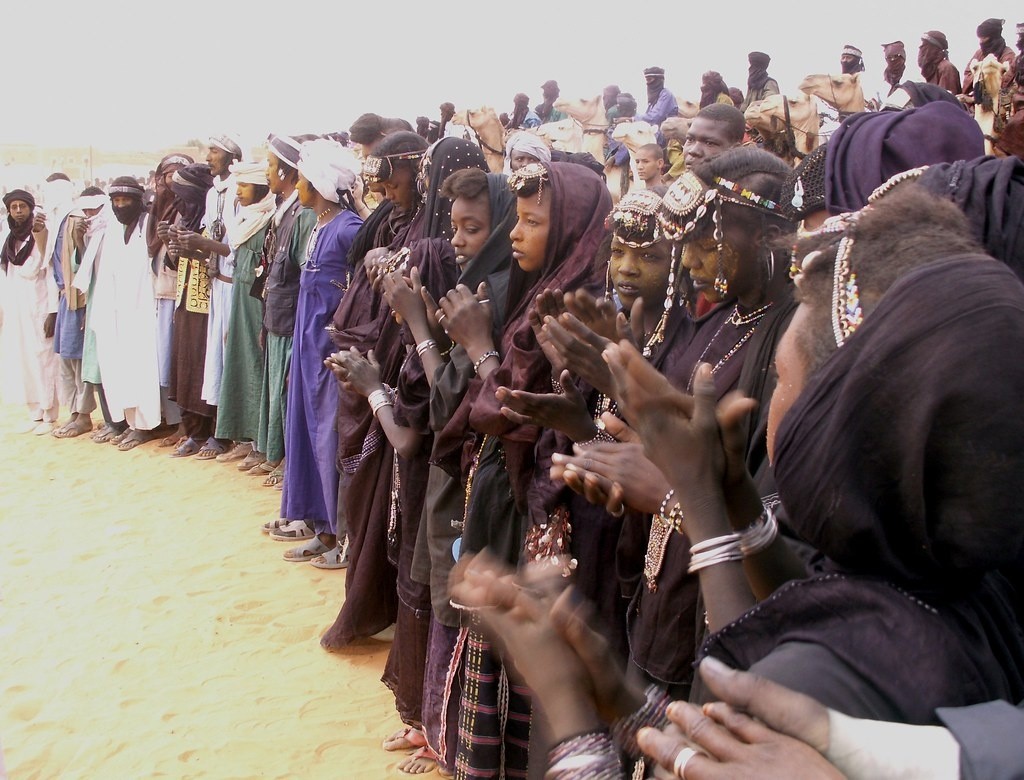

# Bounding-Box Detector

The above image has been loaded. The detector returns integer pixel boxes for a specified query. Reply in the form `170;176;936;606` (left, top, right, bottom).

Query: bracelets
551;378;564;395
416;339;436;356
735;509;778;554
575;429;616;448
369;390;393;417
440;340;454;356
357;205;365;212
474;351;500;376
660;490;684;534
687;533;745;573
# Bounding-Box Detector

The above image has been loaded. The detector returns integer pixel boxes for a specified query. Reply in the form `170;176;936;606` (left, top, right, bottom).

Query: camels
451;104;508;175
552;92;635;206
760;92;820;170
535;117;584;155
744;100;794;169
970;53;1010;157
611;120;659;193
660;116;697;146
674;98;701;118
799;72;866;114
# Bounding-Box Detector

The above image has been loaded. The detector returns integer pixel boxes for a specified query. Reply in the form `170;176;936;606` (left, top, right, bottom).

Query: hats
160;153;195;173
976;18;1005;39
643;66;665;79
228;158;270;186
841;45;863;58
880;40;906;61
296;138;362;203
2;189;35;212
921;31;948;50
171;163;214;192
109;176;145;198
208;134;242;157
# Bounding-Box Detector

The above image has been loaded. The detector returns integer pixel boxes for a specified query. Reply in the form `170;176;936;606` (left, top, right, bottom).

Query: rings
479;299;490;303
438;315;445;323
176;249;178;253
346;373;350;379
673;748;709;780
612;504;623;517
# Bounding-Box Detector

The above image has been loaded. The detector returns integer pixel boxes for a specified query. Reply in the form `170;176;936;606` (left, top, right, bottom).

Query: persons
1;18;1024;780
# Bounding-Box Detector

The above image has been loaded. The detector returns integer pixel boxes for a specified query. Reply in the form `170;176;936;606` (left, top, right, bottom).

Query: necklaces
317;208;330;220
688;304;771;397
594;308;664;419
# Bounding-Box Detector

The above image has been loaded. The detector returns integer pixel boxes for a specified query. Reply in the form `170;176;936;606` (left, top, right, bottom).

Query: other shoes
236;449;267;471
15;420;43;434
31;422;58;435
215;442;253;463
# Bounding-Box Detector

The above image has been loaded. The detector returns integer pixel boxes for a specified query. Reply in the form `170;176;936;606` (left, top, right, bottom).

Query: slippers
308;546;349;570
259;516;316;542
280;535;331;562
382;727;428;752
245;462;276;475
88;421;129;443
109;426;166;451
168;436;206;460
195;435;229;460
396;745;440;777
260;469;284;487
51;418;92;439
